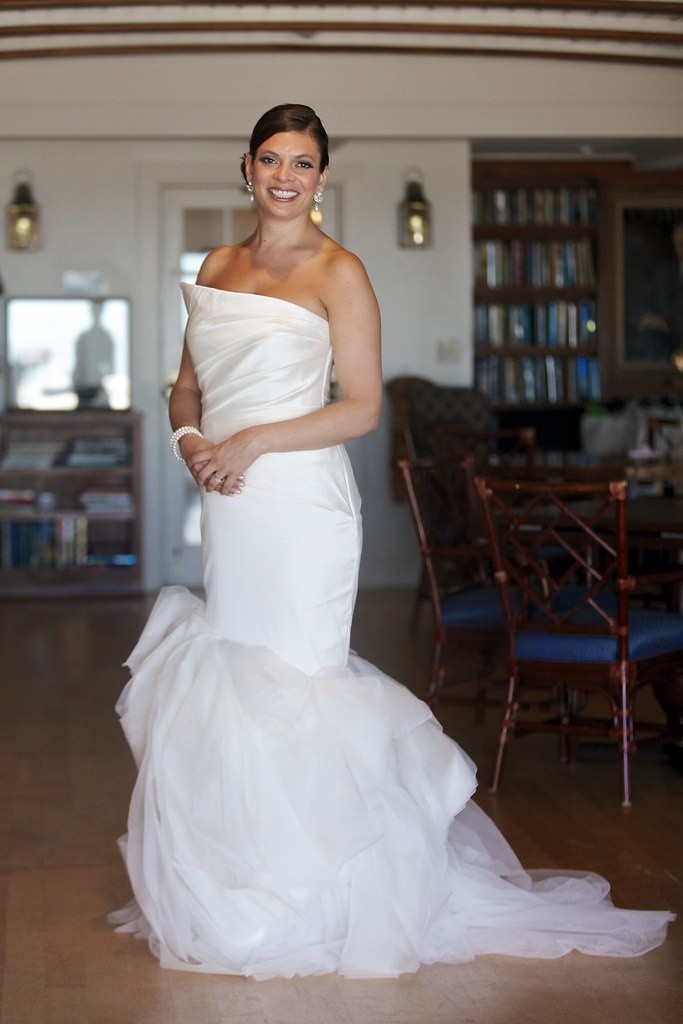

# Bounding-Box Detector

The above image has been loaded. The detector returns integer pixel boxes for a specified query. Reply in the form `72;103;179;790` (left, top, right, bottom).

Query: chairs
391;416;682;811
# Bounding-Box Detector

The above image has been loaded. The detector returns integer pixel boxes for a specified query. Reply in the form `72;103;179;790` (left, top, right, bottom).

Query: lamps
394;183;433;249
7;182;41;252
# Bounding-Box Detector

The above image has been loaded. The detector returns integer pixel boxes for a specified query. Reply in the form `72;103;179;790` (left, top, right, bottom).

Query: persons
167;103;383;965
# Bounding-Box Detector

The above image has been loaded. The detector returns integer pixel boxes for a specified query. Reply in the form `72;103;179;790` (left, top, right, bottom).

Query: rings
214;476;225;483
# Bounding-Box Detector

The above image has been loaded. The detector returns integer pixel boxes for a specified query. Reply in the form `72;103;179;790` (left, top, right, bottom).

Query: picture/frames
613;197;683;373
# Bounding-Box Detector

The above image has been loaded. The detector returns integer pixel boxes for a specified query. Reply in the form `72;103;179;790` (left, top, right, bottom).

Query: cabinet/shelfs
473;156;614;412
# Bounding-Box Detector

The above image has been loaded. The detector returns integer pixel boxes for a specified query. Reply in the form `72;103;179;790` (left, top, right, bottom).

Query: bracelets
170;426;205;462
